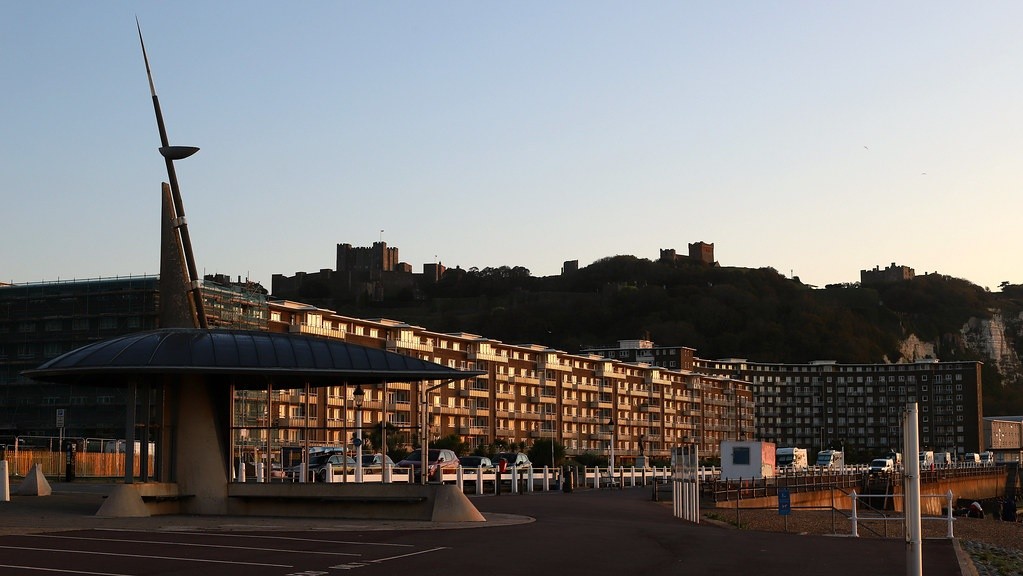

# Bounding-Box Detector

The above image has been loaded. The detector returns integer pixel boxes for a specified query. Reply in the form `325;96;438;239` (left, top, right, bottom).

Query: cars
234;449;533;482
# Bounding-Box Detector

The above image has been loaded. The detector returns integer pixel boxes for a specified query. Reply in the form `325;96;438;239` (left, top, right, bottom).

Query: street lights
607;418;615;485
353;385;365;482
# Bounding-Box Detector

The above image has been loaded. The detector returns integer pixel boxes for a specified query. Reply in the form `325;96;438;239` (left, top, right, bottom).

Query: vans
869;459;894;474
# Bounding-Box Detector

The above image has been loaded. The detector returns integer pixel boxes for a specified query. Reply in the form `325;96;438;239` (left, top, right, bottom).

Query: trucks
933;453;951;469
816;450;842;468
962;453;981;465
919;451;933;470
980;451;993;466
775;447;807;469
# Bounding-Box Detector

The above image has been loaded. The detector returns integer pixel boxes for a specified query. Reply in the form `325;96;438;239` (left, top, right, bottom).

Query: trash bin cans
564;470;574;493
